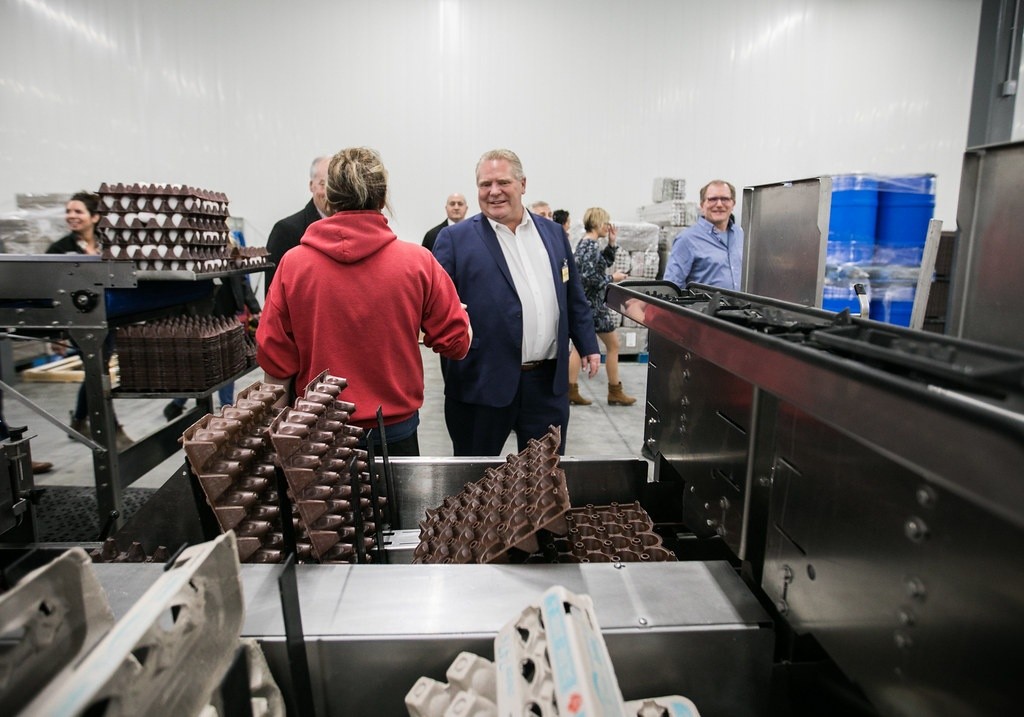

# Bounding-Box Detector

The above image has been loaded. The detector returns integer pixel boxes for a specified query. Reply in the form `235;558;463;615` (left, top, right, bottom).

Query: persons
662;179;743;291
45;193;133;449
256;147;473;456
163;231;261;420
421;149;638;458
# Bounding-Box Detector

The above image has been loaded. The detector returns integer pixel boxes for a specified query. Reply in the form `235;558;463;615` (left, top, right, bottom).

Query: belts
521;359;558;370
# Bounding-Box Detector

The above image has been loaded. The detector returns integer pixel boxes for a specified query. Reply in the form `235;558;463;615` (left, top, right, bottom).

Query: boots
608;381;637;406
568;382;592;405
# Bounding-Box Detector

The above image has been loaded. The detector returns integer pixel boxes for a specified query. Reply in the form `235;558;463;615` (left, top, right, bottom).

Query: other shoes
114;425;135;453
68;410;92;439
32;462;53;474
164;402;186;421
